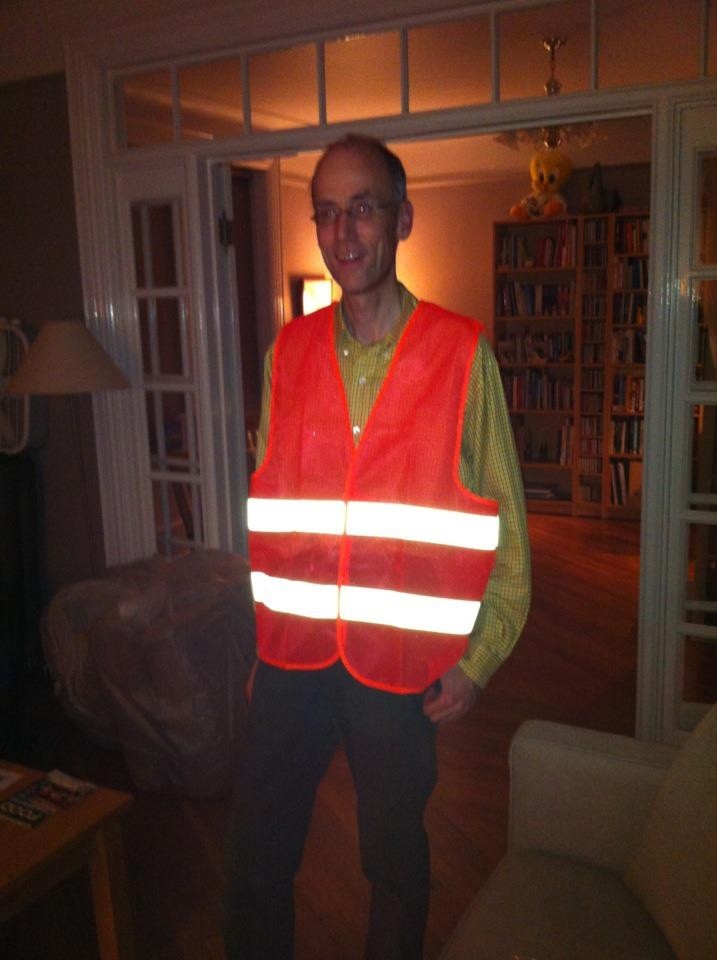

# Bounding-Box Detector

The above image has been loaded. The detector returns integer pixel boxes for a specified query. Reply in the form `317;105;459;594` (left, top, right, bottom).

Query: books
501;369;602;412
496;270;607;316
609;418;644;454
614;331;645;364
584;244;607;265
497;331;574;364
611;369;644;413
495;224;576;269
581;320;606;365
523;484;601;502
616;218;650;253
613;259;647;323
584;218;608;240
610;461;641;506
0;768;97;827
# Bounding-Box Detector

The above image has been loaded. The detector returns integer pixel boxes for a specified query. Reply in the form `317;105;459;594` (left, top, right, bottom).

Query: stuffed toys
510;152;574;221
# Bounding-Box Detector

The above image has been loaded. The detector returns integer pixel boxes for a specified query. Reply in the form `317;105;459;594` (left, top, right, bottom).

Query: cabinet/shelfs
492;205;710;520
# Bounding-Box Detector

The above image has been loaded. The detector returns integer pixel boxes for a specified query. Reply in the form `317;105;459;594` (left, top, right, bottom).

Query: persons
510;415;604;473
223;129;531;960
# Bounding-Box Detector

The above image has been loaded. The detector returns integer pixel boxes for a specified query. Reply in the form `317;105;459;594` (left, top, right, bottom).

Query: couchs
447;703;717;960
41;546;252;808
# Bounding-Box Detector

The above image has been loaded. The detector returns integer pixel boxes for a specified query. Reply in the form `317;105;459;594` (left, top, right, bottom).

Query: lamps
0;320;130;574
490;31;612;152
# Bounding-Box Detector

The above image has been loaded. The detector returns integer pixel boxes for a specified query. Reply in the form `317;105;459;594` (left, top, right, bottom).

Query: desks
0;761;132;960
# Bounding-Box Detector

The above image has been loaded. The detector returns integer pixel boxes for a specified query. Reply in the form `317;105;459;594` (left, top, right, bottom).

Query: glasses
309;201;401;226
